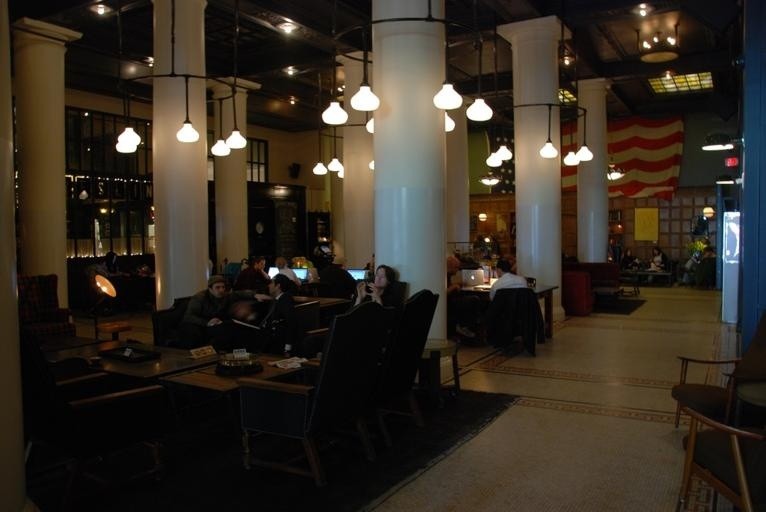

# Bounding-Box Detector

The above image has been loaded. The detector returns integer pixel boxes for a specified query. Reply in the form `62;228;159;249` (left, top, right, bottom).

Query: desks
462;279;562;344
158;352;306;440
64;342;227;378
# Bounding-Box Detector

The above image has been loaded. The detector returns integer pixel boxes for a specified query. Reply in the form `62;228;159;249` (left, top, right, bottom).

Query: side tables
417;338;462;396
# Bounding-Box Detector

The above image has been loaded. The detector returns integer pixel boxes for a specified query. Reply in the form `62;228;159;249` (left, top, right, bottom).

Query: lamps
227;96;248;151
701;133;743;151
465;42;493;122
444;110;455;133
338;163;344;178
210;100;230;157
328;126;340;171
369;160;375;170
322;42;347;125
118;84;142;146
282;24;300;106
351;30;380;111
560;50;573;67
366;117;374;133
174;78;198;143
636;1;677;48
485;105;509;167
608;108;624;180
89;1;112;15
703;207;717;217
434;23;462;110
563;115;579;167
116;90;137;154
478;169;498;185
578;111;592;161
313;131;323;175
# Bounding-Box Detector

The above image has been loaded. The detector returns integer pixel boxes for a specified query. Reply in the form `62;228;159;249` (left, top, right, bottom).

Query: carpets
27;371;521;512
591;299;647;315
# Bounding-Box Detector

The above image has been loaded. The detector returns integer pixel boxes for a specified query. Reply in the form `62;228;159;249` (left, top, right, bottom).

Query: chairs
674;407;766;512
676;307;766;428
365;289;438;448
237;300;398;483
19;256;539;357
562;272;595;317
20;331;164;470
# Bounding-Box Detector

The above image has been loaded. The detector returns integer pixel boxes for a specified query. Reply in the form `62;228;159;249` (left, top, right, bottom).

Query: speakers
290;163;300;179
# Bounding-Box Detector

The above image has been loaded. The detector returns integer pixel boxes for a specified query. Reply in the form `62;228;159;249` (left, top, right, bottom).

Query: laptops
461;270;485;286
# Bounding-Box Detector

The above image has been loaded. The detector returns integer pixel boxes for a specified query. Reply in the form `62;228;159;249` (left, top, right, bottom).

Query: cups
365;278;375;293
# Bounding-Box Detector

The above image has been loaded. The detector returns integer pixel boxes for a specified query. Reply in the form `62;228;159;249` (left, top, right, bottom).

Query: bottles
233;348;247;358
284;344;291;358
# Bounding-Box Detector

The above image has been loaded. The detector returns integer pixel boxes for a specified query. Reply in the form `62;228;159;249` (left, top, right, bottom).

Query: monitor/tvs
268;267;282;279
347;268;368;280
290;267;308;281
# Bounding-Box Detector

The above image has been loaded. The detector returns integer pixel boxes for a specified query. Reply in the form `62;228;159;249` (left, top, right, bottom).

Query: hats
207;276;224;286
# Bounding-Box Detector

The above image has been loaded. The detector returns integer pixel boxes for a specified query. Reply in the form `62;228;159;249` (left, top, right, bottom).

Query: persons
612;240;716;285
447;255;527;303
95;252;130;316
184;257;401;355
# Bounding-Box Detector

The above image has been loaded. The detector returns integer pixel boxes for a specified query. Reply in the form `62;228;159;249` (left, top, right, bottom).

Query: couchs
619;259;680;282
567;262;619;287
